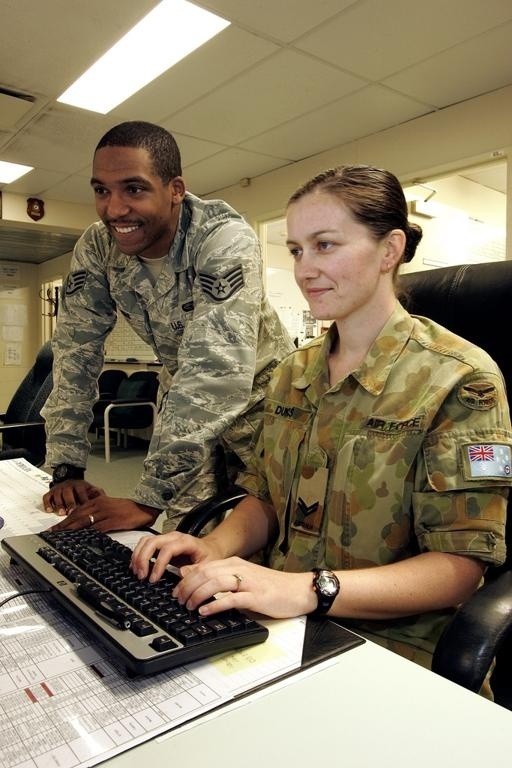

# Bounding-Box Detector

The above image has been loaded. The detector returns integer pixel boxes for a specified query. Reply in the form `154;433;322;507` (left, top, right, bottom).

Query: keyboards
1;525;269;680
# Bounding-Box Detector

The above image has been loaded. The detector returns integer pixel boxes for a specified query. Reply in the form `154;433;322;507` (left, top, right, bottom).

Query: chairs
172;259;511;694
0;337;160;471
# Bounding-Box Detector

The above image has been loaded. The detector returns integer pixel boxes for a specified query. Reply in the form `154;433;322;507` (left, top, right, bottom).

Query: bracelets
53;463;79;483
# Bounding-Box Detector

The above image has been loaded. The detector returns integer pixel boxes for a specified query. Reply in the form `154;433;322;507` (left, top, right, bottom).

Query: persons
37;120;299;567
129;161;510;703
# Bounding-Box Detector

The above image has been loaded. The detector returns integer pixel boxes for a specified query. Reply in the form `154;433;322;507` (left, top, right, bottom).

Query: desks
0;456;512;767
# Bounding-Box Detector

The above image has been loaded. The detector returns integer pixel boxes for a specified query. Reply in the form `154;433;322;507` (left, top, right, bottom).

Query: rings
88;513;96;525
233;573;243;592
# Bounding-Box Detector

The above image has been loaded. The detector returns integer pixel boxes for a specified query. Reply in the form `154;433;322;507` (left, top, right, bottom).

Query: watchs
310;566;340;616
